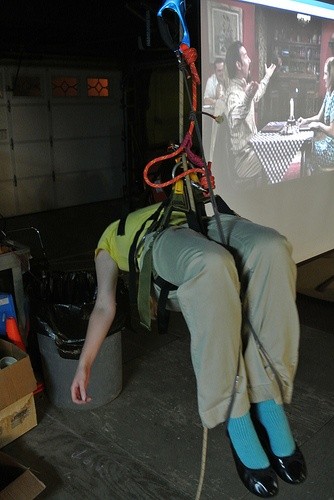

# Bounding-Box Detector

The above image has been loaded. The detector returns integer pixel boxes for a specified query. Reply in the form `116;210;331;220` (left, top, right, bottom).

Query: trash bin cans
26;270;128;412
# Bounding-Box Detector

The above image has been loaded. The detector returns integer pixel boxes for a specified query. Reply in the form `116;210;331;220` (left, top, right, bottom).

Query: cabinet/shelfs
268;42;320;76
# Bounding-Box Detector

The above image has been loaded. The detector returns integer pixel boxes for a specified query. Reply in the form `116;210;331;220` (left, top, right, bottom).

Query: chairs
212;99;232;178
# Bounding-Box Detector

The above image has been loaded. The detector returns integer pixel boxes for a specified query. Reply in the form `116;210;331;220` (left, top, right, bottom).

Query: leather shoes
226;424;279;498
250;404;308;484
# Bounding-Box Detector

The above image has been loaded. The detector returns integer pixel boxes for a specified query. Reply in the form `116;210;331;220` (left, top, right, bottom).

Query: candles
290;98;294;117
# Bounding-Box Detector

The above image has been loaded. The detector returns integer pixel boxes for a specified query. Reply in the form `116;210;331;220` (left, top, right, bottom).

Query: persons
71;201;308;497
203;40;334;191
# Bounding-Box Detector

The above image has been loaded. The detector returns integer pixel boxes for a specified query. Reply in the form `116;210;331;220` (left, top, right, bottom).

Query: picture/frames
207;1;243;64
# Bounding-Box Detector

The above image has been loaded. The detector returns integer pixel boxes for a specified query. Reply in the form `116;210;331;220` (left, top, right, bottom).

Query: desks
1;239;31;339
278;74;317;118
249;122;314;186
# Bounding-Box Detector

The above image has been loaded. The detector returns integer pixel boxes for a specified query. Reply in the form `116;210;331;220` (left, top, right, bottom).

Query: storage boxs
0;294;47;500
